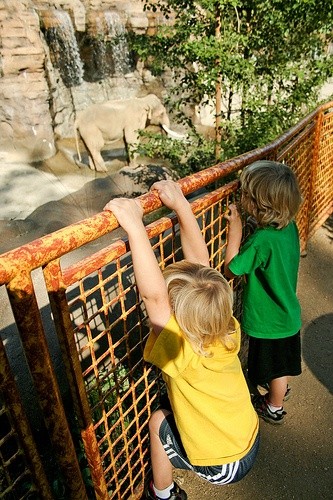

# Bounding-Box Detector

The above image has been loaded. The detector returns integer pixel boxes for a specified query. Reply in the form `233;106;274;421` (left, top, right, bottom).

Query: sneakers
262;381;290;400
251;401;287;425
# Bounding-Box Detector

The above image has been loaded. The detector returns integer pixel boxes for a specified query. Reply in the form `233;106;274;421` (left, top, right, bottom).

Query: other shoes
141;480;188;500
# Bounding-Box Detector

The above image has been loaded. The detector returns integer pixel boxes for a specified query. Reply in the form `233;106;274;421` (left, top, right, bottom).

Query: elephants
74;93;189;171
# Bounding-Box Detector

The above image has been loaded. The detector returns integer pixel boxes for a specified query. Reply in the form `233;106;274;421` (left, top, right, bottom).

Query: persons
223;159;302;426
101;180;260;500
